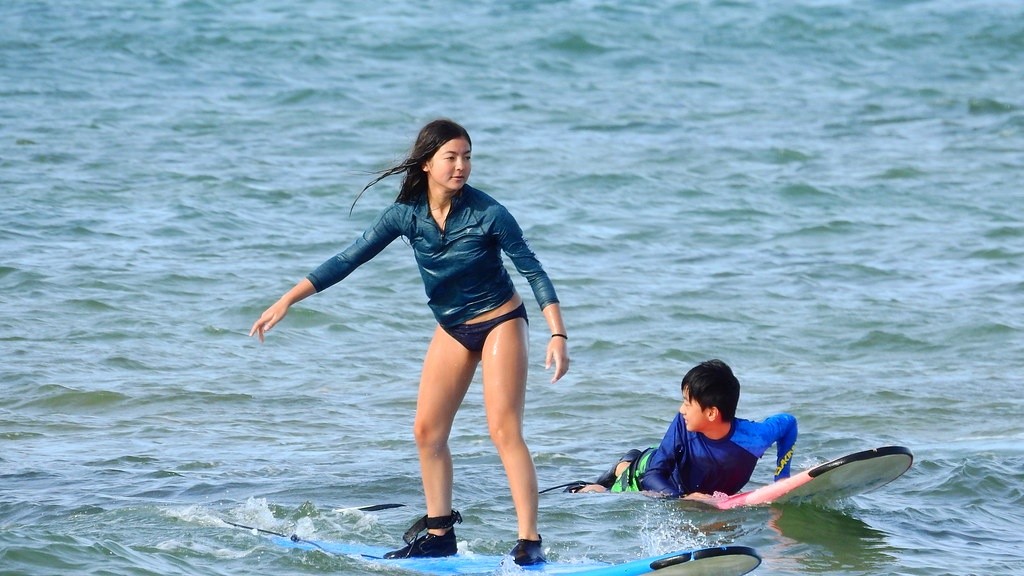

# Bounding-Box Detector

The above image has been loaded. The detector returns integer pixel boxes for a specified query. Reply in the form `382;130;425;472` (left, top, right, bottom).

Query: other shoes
382;528;458;560
597;449;641;488
564;482;587;495
499;533;546;566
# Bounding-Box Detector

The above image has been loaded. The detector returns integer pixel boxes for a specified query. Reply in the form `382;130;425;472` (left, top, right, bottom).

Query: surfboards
699;445;915;508
220;520;762;576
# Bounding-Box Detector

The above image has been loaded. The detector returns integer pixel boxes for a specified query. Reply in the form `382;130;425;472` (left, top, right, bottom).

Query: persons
249;119;570;566
564;360;799;500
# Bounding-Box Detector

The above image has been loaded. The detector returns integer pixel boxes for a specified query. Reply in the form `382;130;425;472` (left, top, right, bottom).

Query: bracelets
551;334;568;340
